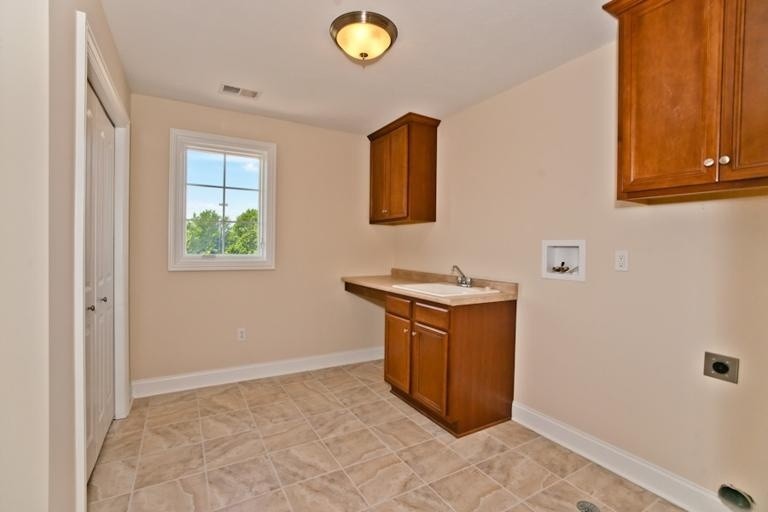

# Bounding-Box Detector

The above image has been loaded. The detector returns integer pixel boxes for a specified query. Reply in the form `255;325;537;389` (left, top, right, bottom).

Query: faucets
450;265;466;284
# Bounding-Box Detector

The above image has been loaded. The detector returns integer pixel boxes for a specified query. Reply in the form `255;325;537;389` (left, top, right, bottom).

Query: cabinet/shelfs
384;291;516;439
367;112;441;225
602;0;768;204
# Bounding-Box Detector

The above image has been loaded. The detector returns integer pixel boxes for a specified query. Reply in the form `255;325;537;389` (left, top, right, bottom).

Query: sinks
391;282;500;297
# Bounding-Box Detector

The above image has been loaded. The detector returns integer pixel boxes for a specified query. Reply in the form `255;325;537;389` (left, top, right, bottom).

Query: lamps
329;10;398;63
